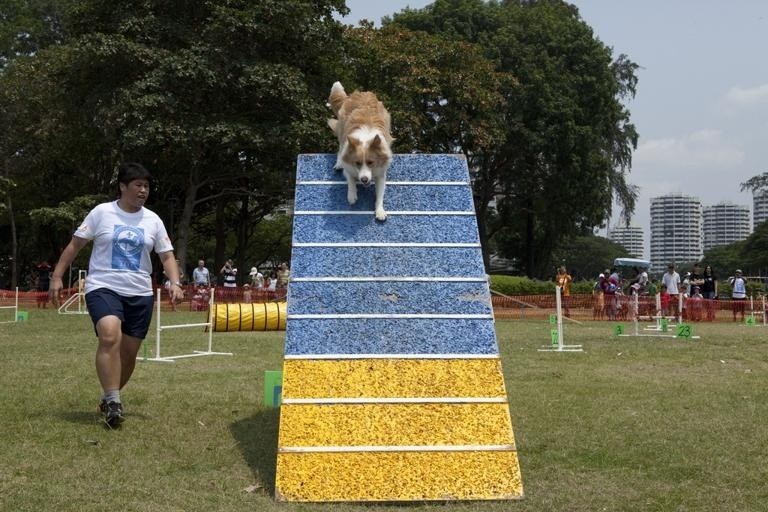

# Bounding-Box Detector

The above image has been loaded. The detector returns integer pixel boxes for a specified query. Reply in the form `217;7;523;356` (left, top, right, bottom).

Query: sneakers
96;397;125;417
105;401;127;426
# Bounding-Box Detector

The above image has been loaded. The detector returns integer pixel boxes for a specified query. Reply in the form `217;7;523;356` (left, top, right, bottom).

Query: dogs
327;80;397;223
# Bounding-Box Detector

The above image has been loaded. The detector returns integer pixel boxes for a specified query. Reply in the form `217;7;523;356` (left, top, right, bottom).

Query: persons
554;262;768;324
46;161;187;428
31;256;291;312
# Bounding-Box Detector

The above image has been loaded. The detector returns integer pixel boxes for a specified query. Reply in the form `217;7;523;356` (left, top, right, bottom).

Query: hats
249;267;258;276
